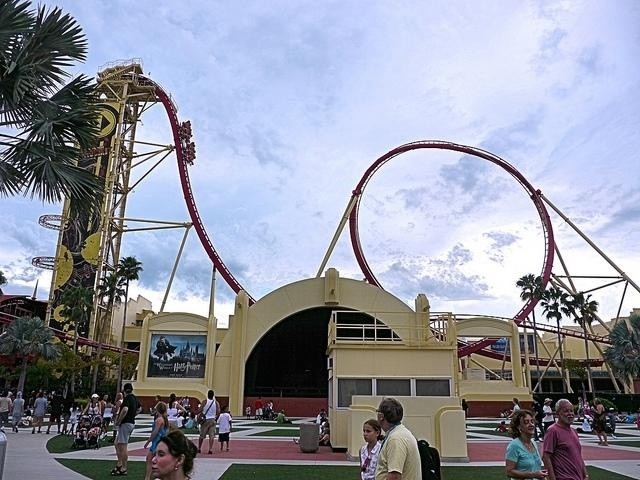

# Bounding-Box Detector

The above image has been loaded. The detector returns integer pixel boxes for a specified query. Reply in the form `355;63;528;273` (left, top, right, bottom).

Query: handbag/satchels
417;440;440;479
198;414;206;425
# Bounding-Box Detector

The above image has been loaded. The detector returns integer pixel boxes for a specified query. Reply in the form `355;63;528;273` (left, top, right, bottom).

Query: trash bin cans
300;422;320;453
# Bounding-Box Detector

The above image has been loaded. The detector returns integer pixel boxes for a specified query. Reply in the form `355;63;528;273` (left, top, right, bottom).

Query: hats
92;394;100;398
125;383;133;390
544;398;552;404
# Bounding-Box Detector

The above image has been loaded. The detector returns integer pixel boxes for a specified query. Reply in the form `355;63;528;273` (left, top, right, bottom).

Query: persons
293;409;330;446
153;336;177;363
359;419;384;480
462;398;469;428
495;396;640;480
375;398;422;480
1;380;232;480
245;399;292;424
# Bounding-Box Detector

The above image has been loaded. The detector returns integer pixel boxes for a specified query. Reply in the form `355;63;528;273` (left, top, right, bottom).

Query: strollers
69;415;104;451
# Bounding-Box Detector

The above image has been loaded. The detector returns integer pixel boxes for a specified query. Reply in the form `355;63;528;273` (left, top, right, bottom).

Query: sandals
110;465;128;476
